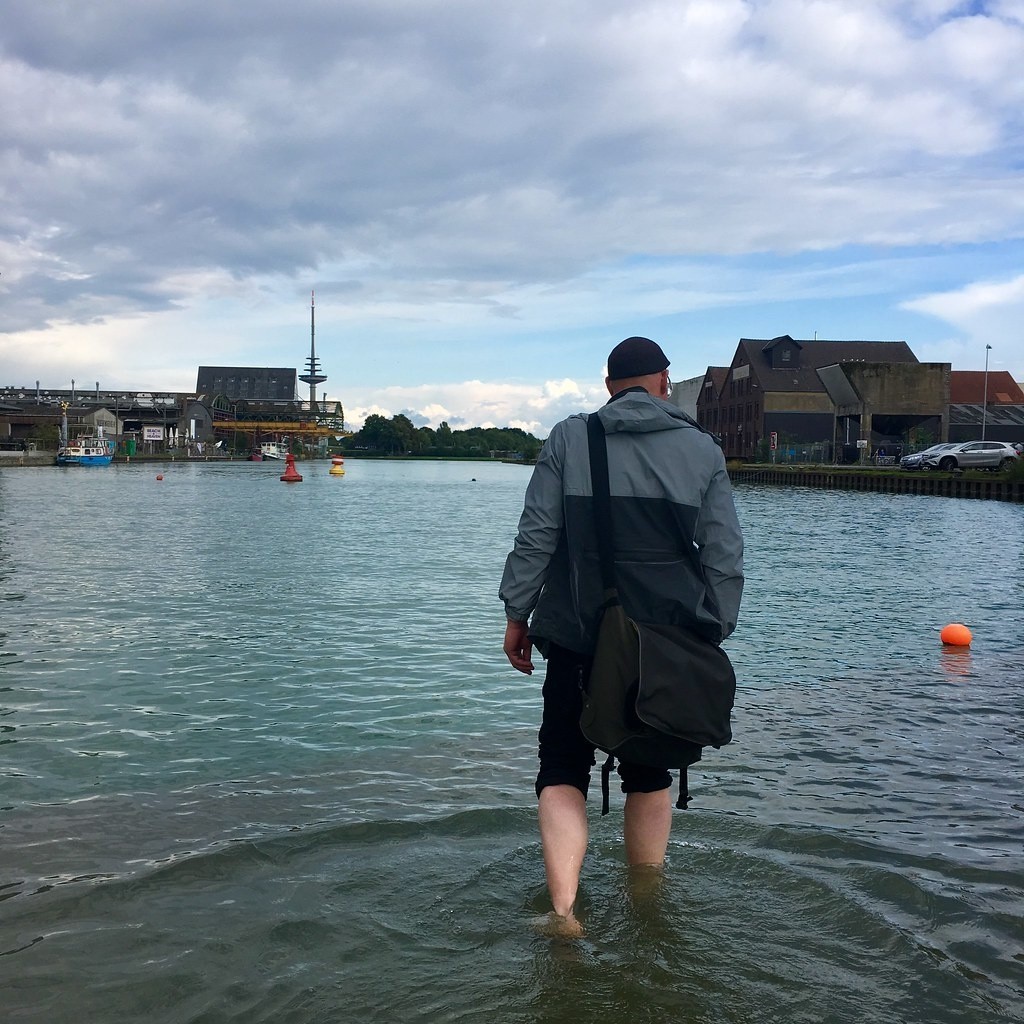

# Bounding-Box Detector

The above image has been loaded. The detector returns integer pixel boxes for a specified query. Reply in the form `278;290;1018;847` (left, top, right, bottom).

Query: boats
55;423;115;467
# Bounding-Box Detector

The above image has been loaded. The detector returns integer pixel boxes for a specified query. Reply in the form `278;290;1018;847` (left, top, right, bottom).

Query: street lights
982;344;992;441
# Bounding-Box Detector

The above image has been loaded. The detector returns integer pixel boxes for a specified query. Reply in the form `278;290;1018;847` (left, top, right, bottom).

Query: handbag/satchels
577;606;736;769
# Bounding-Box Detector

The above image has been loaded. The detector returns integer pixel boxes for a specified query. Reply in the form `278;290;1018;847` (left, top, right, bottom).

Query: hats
607;338;671;381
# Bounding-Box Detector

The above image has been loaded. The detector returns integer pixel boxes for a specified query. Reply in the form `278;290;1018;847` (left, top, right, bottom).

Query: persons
496;334;746;938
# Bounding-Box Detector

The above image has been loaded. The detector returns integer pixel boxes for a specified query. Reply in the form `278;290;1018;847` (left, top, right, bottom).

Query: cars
900;442;951;472
915;440;1024;473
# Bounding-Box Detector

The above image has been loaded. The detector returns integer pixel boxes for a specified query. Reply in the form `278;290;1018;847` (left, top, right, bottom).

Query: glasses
667;376;674;399
